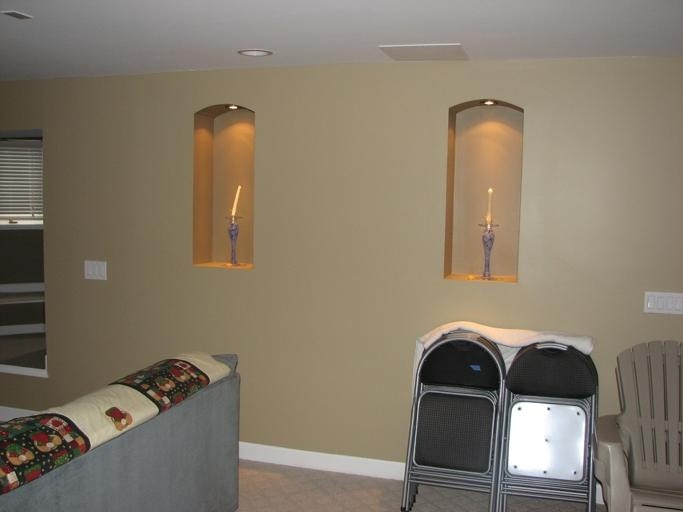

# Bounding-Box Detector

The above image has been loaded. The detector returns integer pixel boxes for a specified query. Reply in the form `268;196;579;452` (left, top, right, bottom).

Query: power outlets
642;290;683;314
83;260;108;280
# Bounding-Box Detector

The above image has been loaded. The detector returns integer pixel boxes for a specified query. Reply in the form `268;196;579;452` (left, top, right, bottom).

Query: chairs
401;331;596;512
593;340;683;512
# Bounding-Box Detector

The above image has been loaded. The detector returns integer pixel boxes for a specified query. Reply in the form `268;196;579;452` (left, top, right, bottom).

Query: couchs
0;352;239;511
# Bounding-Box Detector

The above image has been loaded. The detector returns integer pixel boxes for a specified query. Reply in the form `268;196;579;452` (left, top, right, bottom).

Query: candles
482;184;496;227
228;184;244;220
